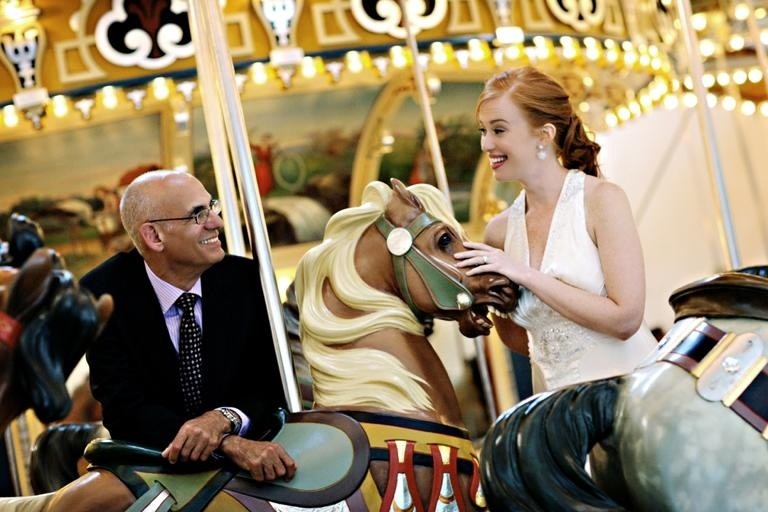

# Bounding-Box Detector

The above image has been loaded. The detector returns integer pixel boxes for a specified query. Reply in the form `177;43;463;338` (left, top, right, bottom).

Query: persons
451;67;660;392
74;169;299;484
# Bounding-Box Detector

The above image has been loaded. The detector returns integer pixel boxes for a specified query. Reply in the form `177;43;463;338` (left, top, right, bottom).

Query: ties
174;293;202;413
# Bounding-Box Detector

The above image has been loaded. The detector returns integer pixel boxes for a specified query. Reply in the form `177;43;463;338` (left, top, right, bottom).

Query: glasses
143;199;221;224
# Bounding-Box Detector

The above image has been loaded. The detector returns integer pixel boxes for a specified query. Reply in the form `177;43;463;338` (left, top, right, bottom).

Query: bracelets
213;407;243;436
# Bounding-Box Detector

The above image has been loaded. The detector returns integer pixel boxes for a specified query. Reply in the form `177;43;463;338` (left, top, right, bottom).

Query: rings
483;256;488;264
